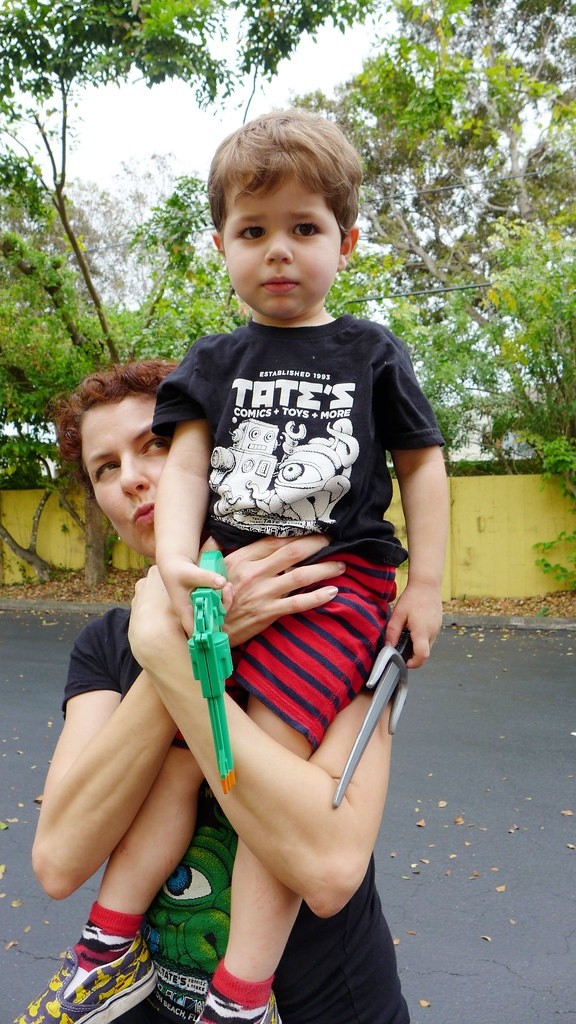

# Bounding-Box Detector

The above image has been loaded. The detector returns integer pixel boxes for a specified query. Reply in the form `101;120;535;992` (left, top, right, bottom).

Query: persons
10;109;447;1024
32;354;410;1024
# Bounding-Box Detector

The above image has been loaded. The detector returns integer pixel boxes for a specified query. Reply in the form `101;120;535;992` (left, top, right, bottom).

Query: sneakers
12;929;157;1024
195;988;281;1024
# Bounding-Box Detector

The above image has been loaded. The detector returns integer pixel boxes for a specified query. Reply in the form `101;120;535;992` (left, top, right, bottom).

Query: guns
191;551;242;794
